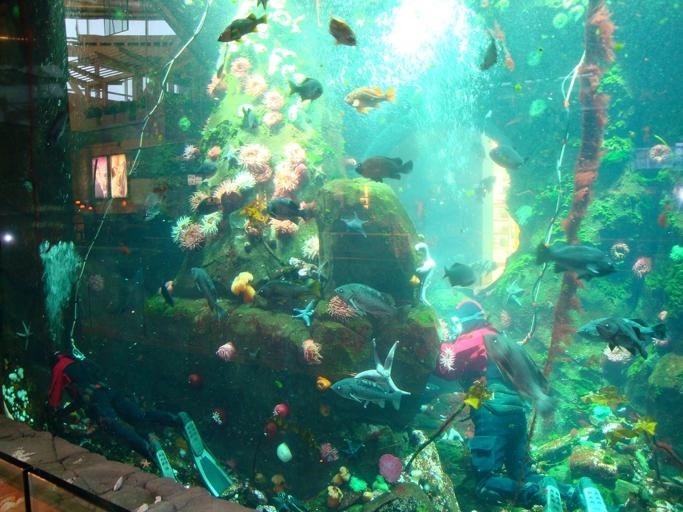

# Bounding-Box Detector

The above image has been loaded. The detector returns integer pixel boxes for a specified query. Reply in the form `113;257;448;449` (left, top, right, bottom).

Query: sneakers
177;412;204;456
147;437;174;478
529;477;563;512
573;477;601;512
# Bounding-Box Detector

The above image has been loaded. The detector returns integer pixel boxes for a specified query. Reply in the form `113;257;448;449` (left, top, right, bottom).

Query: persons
435;298;608;512
43;346;204;471
